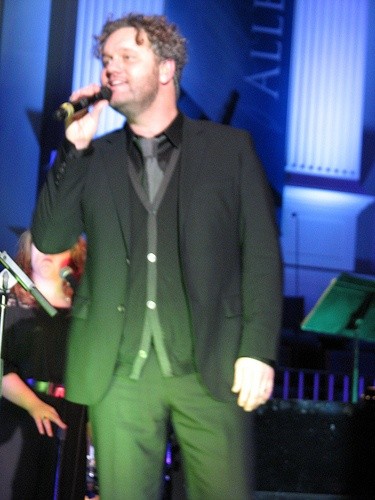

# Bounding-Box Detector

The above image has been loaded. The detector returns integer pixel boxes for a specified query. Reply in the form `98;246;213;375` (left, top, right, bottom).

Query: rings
42;416;48;420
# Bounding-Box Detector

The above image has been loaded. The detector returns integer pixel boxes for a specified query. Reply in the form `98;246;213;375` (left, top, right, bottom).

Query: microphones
54;85;112;122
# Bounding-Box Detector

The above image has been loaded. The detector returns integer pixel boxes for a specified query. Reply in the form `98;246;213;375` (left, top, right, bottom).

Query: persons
30;15;280;500
0;225;90;500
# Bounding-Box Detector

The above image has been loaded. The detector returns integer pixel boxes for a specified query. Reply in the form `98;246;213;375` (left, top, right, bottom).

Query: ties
132;136;164;204
